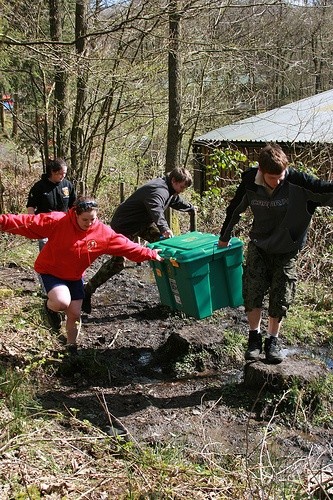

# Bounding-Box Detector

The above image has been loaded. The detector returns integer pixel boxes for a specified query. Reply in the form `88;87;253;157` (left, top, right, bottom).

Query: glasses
75;200;97;209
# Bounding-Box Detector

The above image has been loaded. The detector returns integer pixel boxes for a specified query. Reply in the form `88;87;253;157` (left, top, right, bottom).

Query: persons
27;158;77;294
0;197;164;357
218;144;333;363
82;168;198;314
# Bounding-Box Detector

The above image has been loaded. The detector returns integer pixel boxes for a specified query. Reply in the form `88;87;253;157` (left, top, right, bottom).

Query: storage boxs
146;231;246;320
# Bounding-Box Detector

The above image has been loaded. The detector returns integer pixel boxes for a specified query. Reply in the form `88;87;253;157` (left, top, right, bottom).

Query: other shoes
82;284;91;314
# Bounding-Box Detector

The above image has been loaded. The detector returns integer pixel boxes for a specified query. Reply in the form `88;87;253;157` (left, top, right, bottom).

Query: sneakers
43;300;62;330
67;345;80;363
263;335;283;364
244;330;263;360
